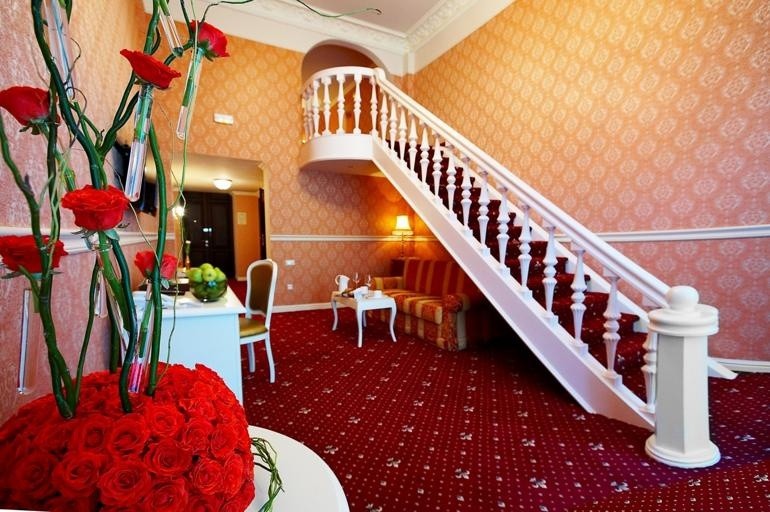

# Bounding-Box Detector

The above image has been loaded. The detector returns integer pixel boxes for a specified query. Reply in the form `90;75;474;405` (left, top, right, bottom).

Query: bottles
44;0;75;103
125;1;206;202
15;244;155;396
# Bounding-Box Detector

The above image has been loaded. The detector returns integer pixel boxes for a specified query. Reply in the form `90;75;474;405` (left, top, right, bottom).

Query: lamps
392;214;414;257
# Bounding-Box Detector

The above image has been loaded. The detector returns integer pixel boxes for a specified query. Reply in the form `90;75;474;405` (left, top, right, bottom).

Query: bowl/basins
190;280;227;302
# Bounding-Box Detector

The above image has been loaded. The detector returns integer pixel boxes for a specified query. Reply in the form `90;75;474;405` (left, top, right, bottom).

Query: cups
354;271;372;302
374;290;381;299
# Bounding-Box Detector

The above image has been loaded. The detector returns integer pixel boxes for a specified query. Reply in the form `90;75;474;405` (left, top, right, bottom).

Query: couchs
367;255;483;353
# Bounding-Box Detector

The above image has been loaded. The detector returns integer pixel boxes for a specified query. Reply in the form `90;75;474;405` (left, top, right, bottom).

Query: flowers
0;0;386;512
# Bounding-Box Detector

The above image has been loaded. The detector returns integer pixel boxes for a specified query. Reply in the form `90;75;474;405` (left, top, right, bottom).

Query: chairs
240;257;280;385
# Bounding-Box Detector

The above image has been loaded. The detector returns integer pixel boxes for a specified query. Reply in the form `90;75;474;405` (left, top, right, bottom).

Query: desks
120;265;247;422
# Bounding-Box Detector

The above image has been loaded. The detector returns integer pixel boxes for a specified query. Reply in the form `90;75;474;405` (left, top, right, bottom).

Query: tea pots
336;275;350;294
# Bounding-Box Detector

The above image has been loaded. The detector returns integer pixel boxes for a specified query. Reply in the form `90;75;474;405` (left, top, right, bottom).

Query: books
137;267;190;296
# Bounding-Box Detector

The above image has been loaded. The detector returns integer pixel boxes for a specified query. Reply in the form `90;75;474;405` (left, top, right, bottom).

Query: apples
188;263;226;299
